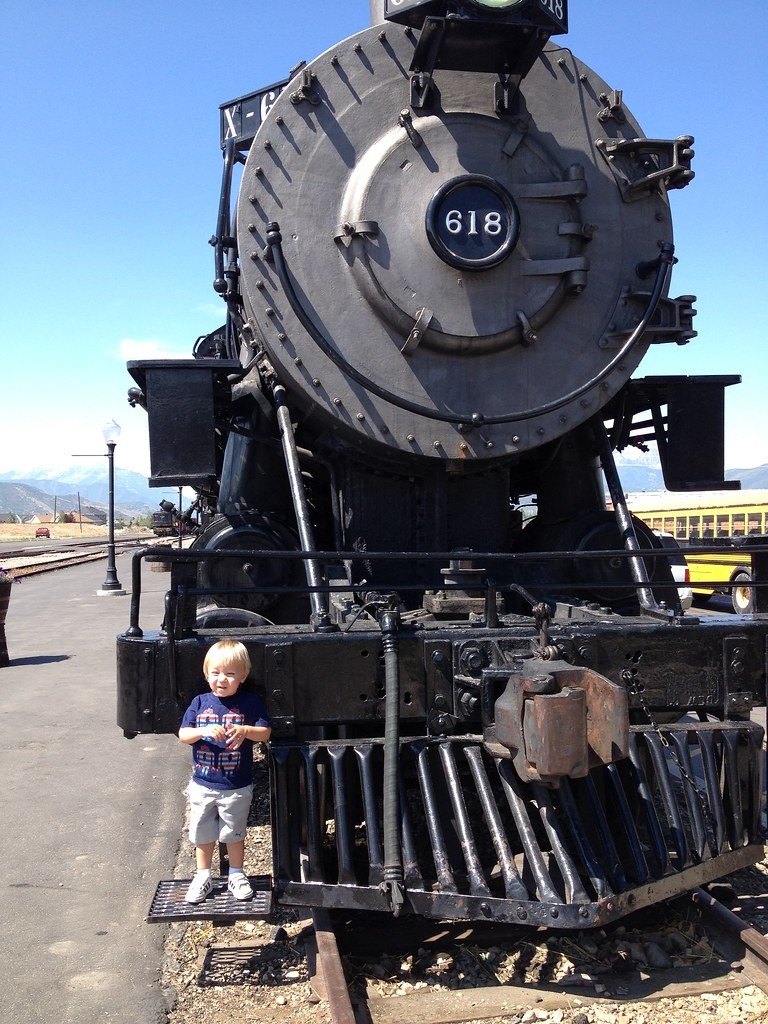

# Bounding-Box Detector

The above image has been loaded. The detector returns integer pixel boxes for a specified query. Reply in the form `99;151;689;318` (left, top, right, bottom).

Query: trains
115;0;768;927
153;509;197;537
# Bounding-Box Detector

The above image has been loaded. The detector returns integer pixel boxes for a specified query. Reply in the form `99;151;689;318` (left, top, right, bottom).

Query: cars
647;527;693;614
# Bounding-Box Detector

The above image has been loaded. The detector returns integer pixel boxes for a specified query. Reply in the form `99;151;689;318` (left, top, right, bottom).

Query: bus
619;489;768;615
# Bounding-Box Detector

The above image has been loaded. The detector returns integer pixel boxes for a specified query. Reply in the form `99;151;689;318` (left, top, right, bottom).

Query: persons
178;640;271;904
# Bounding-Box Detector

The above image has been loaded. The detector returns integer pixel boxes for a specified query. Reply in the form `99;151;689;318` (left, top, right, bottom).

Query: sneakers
228;872;253;899
185;874;213;903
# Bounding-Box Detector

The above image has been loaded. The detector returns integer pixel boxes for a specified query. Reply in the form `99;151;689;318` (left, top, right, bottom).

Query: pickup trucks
35;528;50;538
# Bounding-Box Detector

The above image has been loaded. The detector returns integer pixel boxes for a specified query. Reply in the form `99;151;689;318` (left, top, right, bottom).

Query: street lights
195;492;201;524
96;419;126;596
